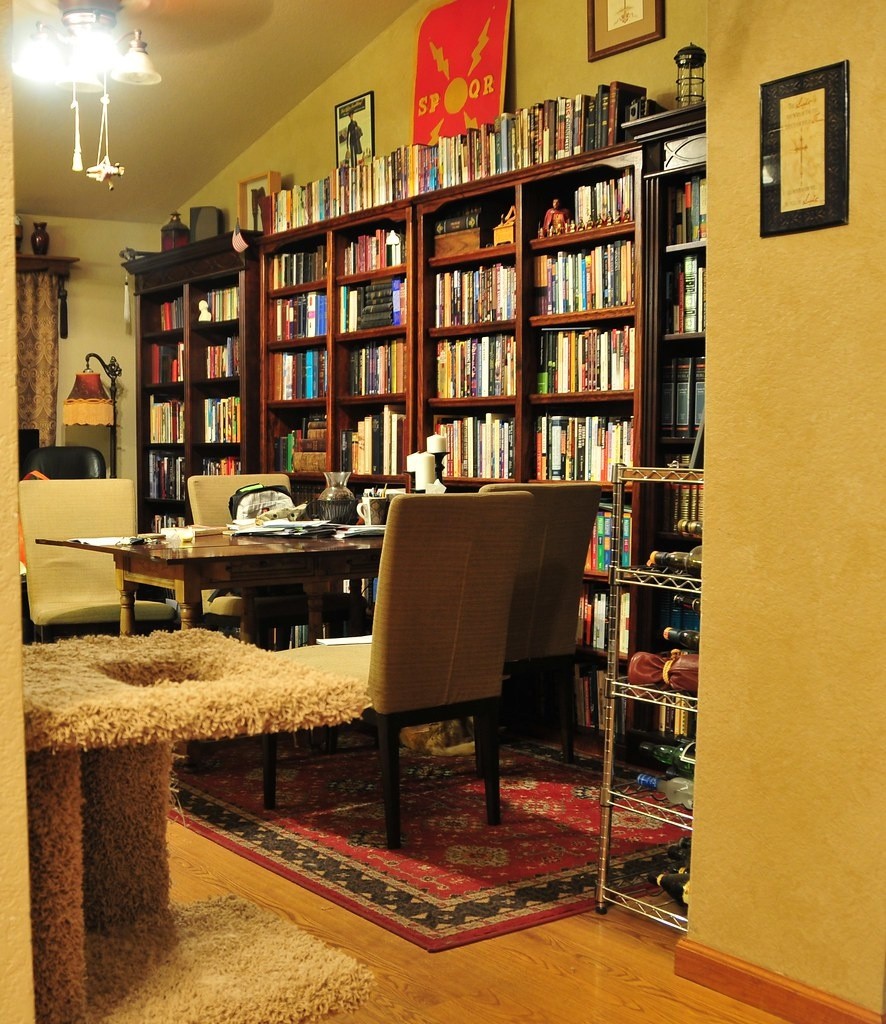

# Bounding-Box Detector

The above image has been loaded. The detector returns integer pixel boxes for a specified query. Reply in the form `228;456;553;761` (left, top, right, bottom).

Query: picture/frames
760;60;850;237
587;0;666;62
237;171;282;229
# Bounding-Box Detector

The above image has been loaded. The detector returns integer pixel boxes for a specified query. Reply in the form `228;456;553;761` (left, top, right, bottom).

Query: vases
30;222;49;255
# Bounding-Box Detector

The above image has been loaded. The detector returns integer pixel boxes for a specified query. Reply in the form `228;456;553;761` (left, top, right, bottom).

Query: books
149;81;708;735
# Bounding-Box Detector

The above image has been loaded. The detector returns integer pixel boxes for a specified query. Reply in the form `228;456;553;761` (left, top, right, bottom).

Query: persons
346;110;363;168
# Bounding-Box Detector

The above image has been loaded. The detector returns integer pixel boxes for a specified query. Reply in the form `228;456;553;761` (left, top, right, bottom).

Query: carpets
165;720;693;952
85;896;379;1024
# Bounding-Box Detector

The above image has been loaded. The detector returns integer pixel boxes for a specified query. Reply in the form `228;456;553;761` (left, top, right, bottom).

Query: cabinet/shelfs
254;199;417;494
412;141;644;761
120;229;263;637
622;100;705;768
595;462;704;933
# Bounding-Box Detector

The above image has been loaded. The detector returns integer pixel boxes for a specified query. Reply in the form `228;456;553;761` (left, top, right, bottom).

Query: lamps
13;0;162;191
63;353;122;479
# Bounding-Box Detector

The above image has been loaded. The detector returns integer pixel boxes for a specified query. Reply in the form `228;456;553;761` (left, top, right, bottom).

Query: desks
36;534;384;774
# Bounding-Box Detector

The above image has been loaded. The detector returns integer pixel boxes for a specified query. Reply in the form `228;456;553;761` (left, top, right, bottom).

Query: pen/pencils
363;483;388;497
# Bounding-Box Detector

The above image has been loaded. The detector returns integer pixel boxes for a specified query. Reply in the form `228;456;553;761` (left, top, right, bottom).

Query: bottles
677;518;703;537
674;594;700;617
663;627;700;650
639;742;695;776
636;773;694;808
648;837;691;909
649;545;703;577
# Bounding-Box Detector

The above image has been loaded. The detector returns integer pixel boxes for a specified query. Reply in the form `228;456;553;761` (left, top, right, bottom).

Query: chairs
18;478;177;645
262;491;533;851
188;473;368;748
19;446;106;481
450;482;602;780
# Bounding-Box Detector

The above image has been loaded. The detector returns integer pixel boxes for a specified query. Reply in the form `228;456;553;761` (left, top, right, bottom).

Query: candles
407;432;446;491
